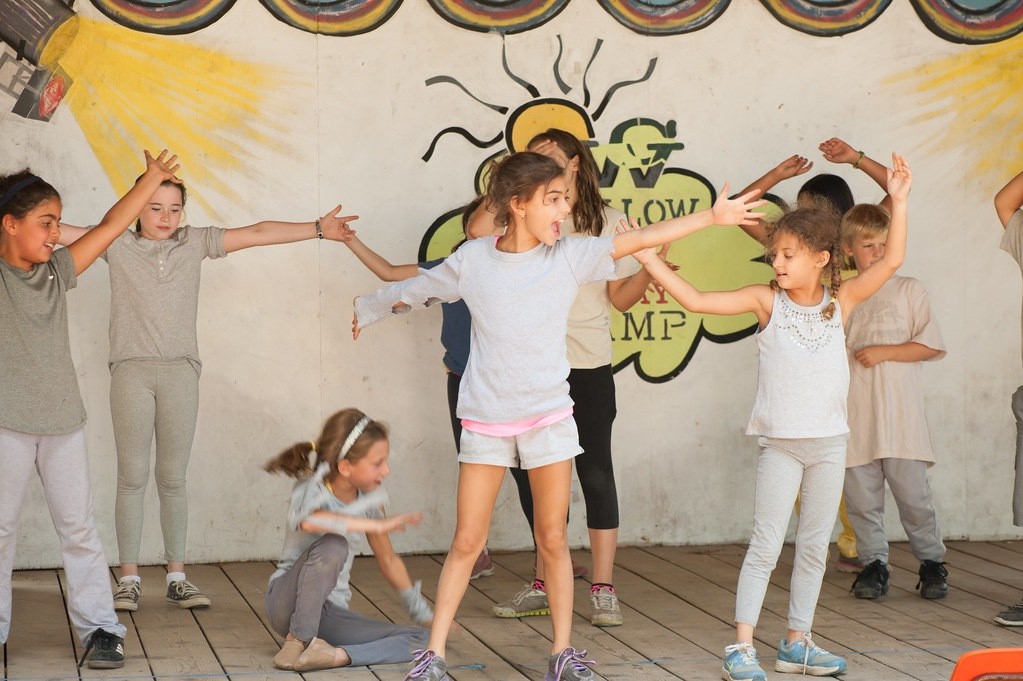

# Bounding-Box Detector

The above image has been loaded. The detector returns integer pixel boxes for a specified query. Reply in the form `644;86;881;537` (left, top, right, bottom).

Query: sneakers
469;550;494;580
166;580;212;608
545;647;597;681
849;558;889;598
774;633;847;676
721;642;767;681
916;560;949;598
404;649;448;681
112;580;143;611
493;582;551;617
589;588;623;626
79;627;125;669
533;554;588;577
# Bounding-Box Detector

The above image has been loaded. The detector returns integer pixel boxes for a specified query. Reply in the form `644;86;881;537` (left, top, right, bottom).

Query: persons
351;152;766;681
616;152;914;681
734;136;895;574
0;147;187;671
261;408;435;673
342;195;592;577
56;175;360;609
993;170;1023;627
463;130;679;627
834;203;951;602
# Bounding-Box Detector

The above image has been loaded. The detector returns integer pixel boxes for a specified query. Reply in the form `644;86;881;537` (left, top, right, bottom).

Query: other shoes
994;599;1023;626
294;636;337;671
835;552;892;572
274;633;305;669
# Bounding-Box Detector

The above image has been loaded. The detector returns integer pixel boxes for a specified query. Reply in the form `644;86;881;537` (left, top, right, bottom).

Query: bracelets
852;151;864;169
314;219;324;240
